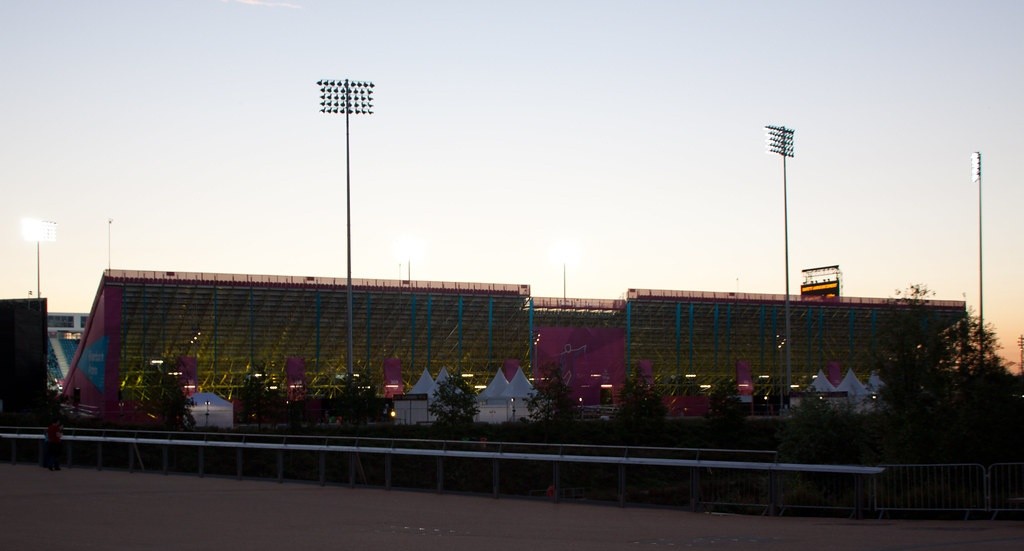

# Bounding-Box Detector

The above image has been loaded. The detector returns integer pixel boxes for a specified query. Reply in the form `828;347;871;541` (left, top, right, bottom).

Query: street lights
204;401;211;426
970;150;985;367
315;77;374;388
766;123;797;412
23;217;57;300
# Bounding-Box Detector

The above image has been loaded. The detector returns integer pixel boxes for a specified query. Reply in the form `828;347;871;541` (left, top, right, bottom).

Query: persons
44;418;63;471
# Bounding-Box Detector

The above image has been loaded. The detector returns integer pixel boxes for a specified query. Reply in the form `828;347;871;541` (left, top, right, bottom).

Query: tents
184;393;233;427
471;366;546;421
799;367;888;414
406;367;465;424
